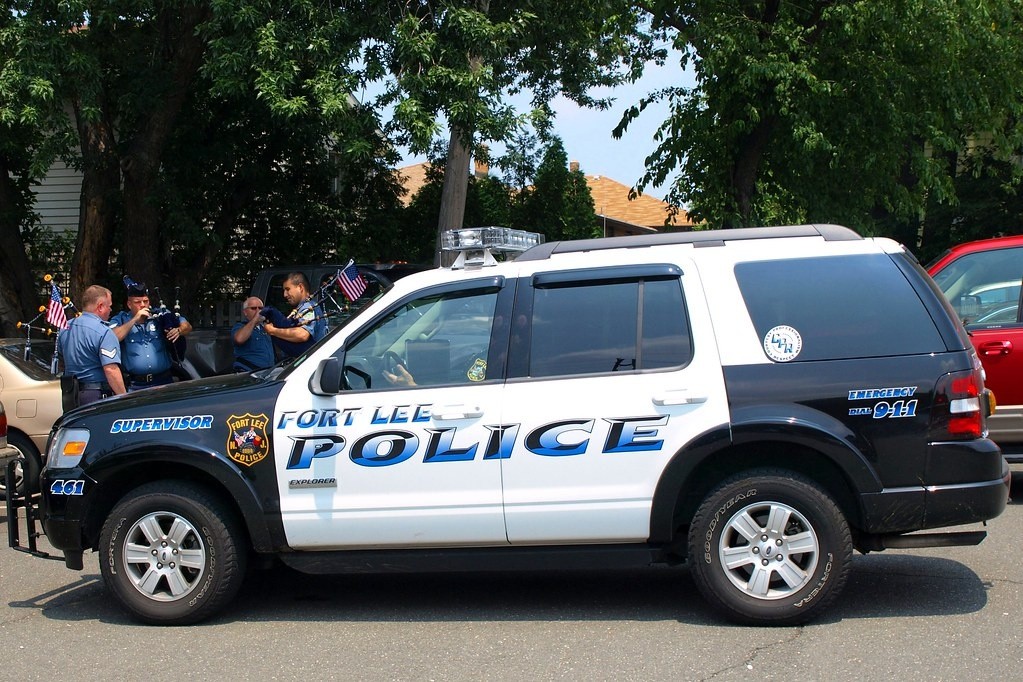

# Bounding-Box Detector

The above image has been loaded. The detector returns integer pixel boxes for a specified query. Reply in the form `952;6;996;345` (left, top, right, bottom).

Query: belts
79;382;103;390
129;374;171;383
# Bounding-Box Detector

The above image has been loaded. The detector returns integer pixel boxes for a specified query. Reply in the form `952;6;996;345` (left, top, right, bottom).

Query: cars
0;335;208;502
244;260;496;382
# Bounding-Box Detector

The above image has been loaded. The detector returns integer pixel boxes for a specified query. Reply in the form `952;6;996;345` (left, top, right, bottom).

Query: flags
334;260;368;303
48;285;70;330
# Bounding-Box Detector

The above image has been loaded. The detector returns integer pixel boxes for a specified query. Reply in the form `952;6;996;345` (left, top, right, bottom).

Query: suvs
32;221;1013;626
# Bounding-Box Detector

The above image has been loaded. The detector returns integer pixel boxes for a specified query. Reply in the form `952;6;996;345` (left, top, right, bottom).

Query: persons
230;296;275;373
264;272;328;359
380;298;497;387
56;285;127;409
108;284;193;392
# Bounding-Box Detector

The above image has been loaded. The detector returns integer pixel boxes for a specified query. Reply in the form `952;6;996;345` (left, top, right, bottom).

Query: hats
128;284;146;297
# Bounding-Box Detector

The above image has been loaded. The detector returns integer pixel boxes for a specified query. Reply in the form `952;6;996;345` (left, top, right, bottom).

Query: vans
919;233;1023;479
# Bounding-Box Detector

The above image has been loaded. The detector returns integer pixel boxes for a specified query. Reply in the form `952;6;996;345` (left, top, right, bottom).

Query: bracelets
176;327;181;336
